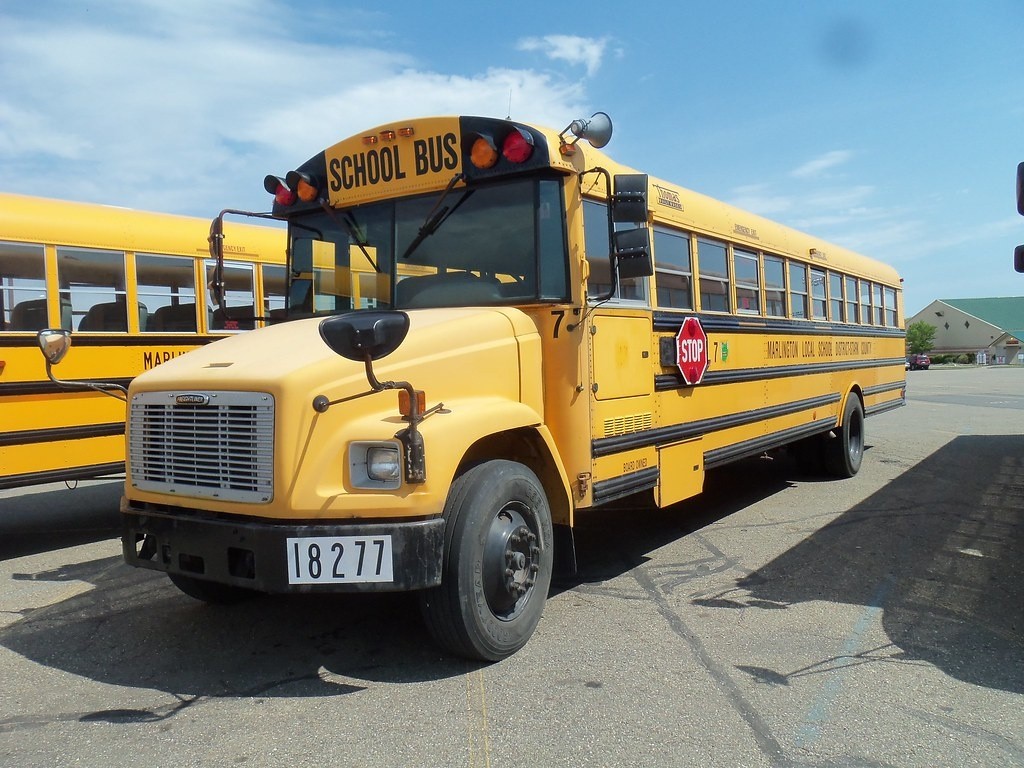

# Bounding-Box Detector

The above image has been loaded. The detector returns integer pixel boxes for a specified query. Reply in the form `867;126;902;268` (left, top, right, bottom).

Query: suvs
910;355;930;370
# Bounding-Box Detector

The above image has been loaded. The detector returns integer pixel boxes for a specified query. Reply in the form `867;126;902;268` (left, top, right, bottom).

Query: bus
1;193;525;489
117;111;908;665
584;254;795;317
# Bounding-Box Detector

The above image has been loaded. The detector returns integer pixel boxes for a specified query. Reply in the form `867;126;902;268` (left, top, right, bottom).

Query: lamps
1019;346;1021;349
1004;347;1006;349
1010;337;1014;340
991;336;993;338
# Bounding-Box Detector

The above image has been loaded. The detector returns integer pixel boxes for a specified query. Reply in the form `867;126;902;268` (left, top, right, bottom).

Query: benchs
153;304;212;332
214;306;270;330
480;277;506;301
88;301;148;332
269;309;318;326
9;299;73;331
316;310;338;316
396;272;485;309
